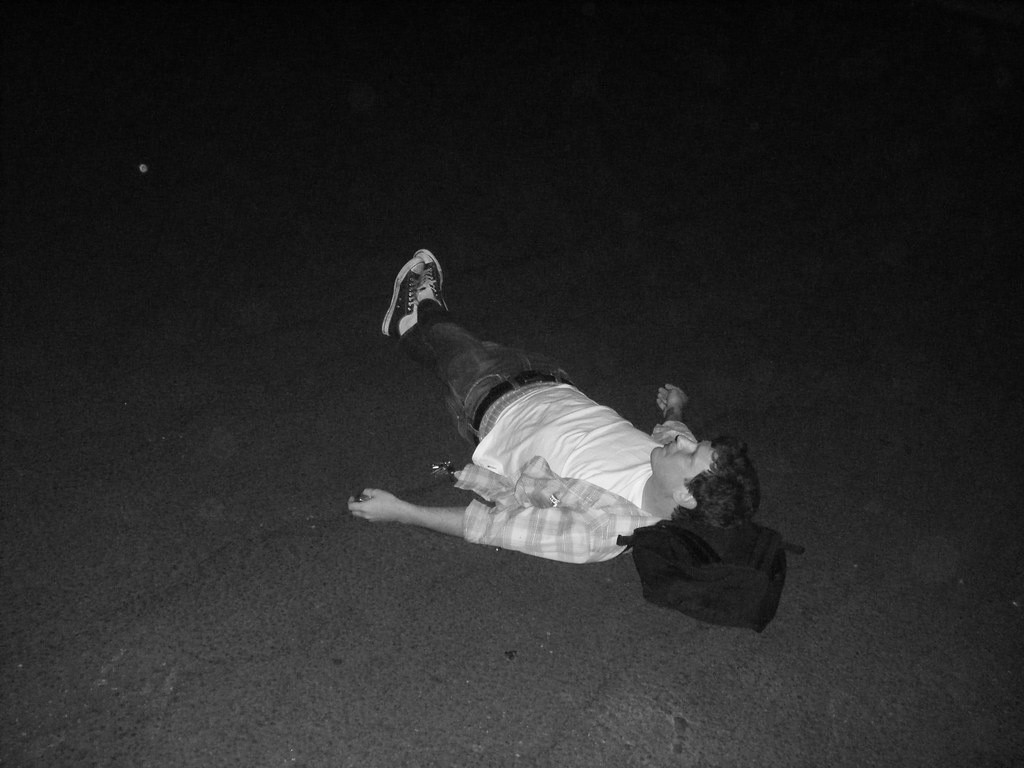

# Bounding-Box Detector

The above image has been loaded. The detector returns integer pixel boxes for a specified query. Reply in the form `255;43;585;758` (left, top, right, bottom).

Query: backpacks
616;520;806;633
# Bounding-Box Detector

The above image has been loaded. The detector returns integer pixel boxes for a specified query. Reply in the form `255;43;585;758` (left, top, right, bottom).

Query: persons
345;249;762;563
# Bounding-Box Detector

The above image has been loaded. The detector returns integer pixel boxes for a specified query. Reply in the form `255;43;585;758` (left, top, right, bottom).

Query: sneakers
411;248;449;314
380;257;425;340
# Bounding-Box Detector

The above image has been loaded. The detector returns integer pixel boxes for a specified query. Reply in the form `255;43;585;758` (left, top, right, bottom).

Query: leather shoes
473;372;572;446
